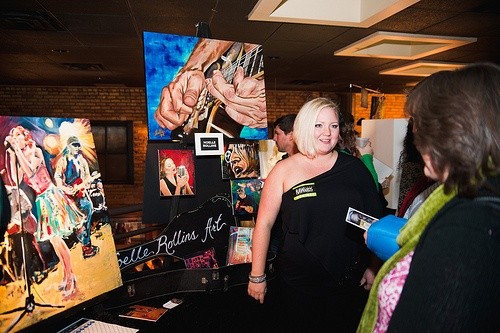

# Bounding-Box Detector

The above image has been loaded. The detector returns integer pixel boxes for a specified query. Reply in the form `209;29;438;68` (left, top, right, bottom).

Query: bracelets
249;273;266;283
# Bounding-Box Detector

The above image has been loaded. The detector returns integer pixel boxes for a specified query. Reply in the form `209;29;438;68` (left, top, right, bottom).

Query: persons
247;63;500;333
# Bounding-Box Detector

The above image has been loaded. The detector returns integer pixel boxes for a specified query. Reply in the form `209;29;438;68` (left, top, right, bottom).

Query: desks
15;261;282;333
